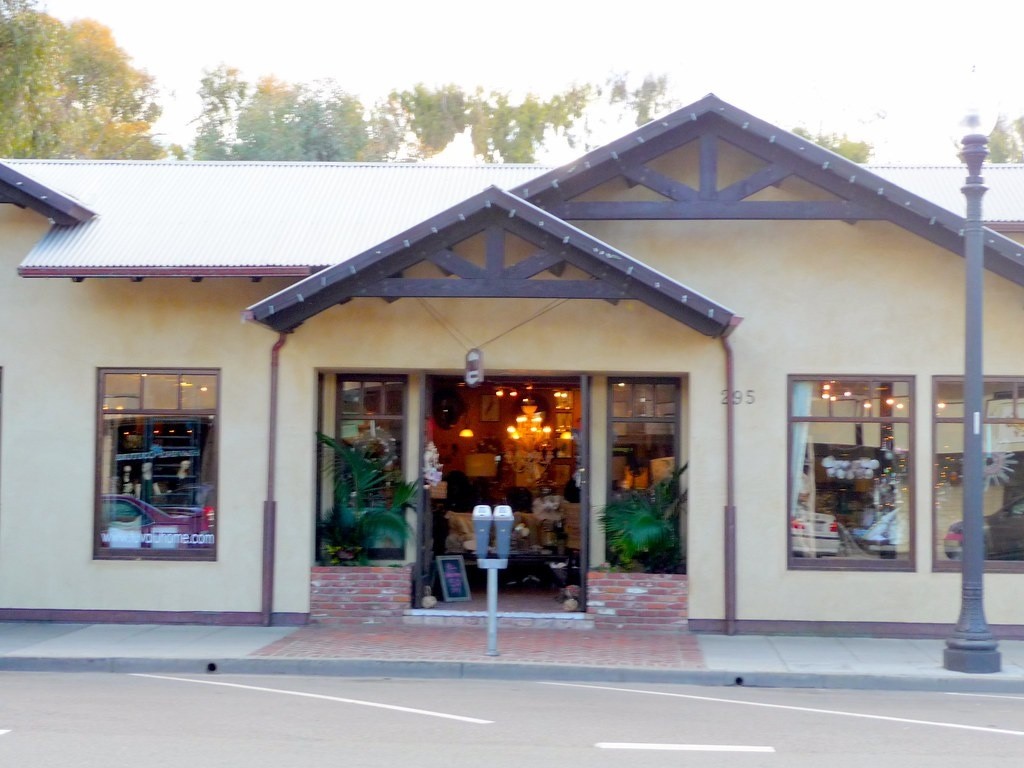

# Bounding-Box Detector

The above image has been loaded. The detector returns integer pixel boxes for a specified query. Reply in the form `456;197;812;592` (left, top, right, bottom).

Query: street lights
939;47;1005;673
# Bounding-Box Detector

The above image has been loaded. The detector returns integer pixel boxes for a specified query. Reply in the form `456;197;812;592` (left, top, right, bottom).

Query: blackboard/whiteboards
436;555;472;601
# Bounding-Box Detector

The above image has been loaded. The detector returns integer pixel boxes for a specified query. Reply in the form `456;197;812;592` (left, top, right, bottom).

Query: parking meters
472;505;515;656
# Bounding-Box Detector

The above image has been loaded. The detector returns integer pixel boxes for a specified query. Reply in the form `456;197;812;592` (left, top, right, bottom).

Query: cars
100;493;202;549
943;494;1023;560
790;500;839;556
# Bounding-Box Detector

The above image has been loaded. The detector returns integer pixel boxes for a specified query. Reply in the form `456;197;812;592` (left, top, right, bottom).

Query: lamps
560;427;572;439
459;418;473;437
507;379;551;472
864;399;871;408
464;349;486;388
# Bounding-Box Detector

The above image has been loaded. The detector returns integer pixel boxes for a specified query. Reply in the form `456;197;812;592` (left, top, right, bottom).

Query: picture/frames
479;394;501;422
435;555;472;603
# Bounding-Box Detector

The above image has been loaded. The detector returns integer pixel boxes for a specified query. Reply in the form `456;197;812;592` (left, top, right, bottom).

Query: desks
444;552;573;590
